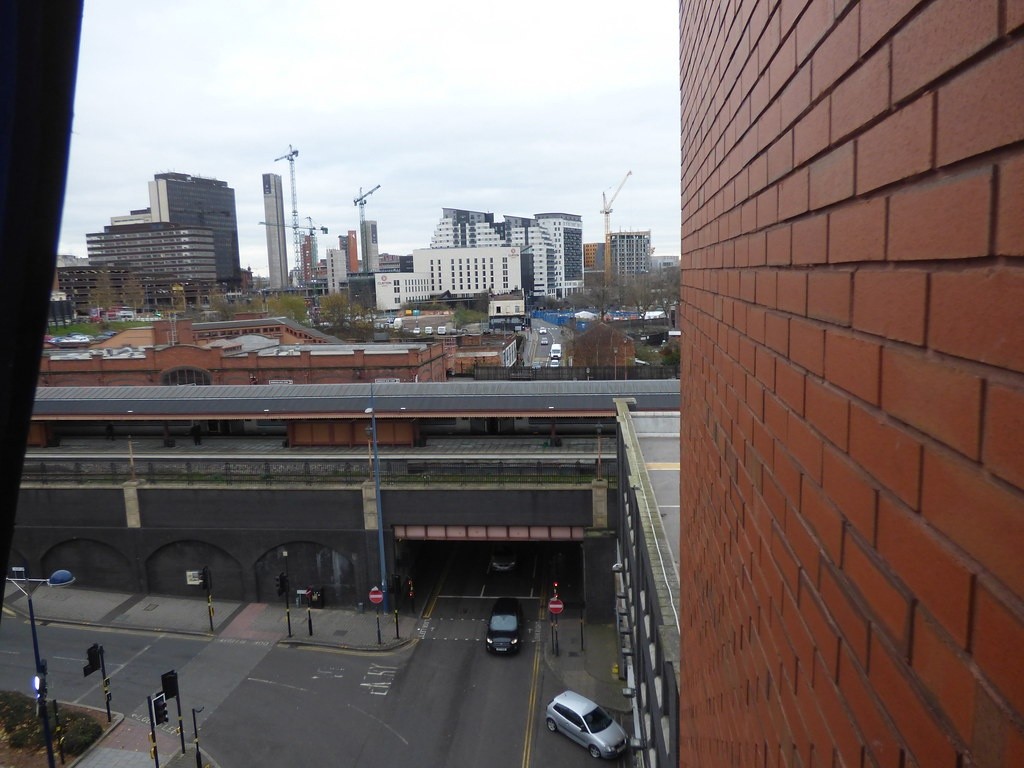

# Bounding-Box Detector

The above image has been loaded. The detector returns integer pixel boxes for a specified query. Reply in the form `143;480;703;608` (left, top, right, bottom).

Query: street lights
5;566;77;766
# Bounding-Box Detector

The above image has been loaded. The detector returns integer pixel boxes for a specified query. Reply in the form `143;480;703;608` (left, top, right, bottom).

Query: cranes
259;144;328;289
354;183;383;273
600;169;634;232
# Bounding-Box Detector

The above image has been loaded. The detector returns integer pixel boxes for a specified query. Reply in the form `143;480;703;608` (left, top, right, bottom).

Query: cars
539;325;545;334
485;597;523;656
550;360;560;367
544;691;628;759
540;337;548;345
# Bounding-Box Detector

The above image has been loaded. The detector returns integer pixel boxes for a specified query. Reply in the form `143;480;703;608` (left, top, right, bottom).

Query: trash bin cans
308;584;325;609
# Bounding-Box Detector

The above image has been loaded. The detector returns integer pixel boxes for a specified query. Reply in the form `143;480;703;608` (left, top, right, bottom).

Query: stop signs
369;589;383;604
548;598;563;614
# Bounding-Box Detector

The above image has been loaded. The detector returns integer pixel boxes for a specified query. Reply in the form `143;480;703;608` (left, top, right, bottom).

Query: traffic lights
407;576;415;599
552;582;559;589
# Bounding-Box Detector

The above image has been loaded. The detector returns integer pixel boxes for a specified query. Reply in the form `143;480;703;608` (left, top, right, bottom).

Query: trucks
550;343;562;360
